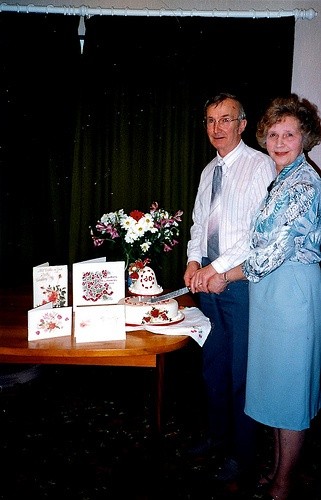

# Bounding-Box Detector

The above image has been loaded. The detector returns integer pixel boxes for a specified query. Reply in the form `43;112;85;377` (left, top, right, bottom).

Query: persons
208;94;321;500
184;92;278;482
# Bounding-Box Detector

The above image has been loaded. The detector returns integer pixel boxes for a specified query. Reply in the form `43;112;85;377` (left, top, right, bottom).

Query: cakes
117;296;179;325
129;260;163;295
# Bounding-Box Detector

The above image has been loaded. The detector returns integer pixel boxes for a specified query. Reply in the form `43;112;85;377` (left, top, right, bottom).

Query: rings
199;282;202;285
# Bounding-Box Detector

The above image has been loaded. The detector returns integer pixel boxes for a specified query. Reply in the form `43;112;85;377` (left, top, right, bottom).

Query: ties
206;163;219;262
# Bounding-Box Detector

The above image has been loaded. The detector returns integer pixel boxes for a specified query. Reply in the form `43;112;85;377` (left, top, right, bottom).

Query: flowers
88;201;183;271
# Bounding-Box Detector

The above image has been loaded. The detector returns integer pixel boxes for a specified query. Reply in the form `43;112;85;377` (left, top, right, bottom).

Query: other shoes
254;470;291;500
192;462;249;483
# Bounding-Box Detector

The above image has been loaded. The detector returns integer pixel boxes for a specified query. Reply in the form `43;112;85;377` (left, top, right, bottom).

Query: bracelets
224;273;230;283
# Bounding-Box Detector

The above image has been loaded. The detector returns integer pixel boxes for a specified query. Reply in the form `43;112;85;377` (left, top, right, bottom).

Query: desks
0;289;197;430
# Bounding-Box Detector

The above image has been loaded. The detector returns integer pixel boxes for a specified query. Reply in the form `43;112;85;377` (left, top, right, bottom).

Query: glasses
203;118;240;127
